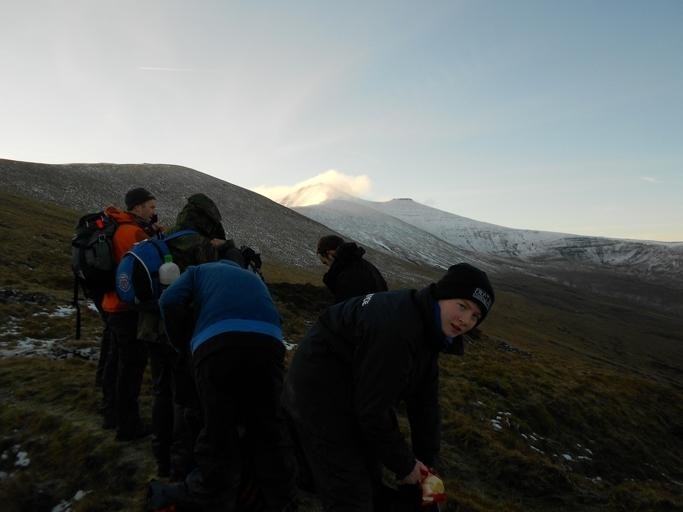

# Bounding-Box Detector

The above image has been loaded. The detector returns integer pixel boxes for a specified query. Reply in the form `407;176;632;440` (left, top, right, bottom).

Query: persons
96;187;286;512
317;235;388;305
289;263;495;512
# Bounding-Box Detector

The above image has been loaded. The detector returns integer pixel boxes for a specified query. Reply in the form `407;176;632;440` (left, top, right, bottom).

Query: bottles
160;255;181;287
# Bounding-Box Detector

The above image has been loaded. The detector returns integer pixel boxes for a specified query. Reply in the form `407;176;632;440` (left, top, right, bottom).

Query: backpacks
67;208;120;305
111;229;199;313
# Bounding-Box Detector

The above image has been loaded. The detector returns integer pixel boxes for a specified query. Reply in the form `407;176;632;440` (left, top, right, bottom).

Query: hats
434;261;495;329
123;185;156;210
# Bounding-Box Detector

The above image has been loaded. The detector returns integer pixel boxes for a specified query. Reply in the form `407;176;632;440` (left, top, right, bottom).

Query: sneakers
114;417;155;445
